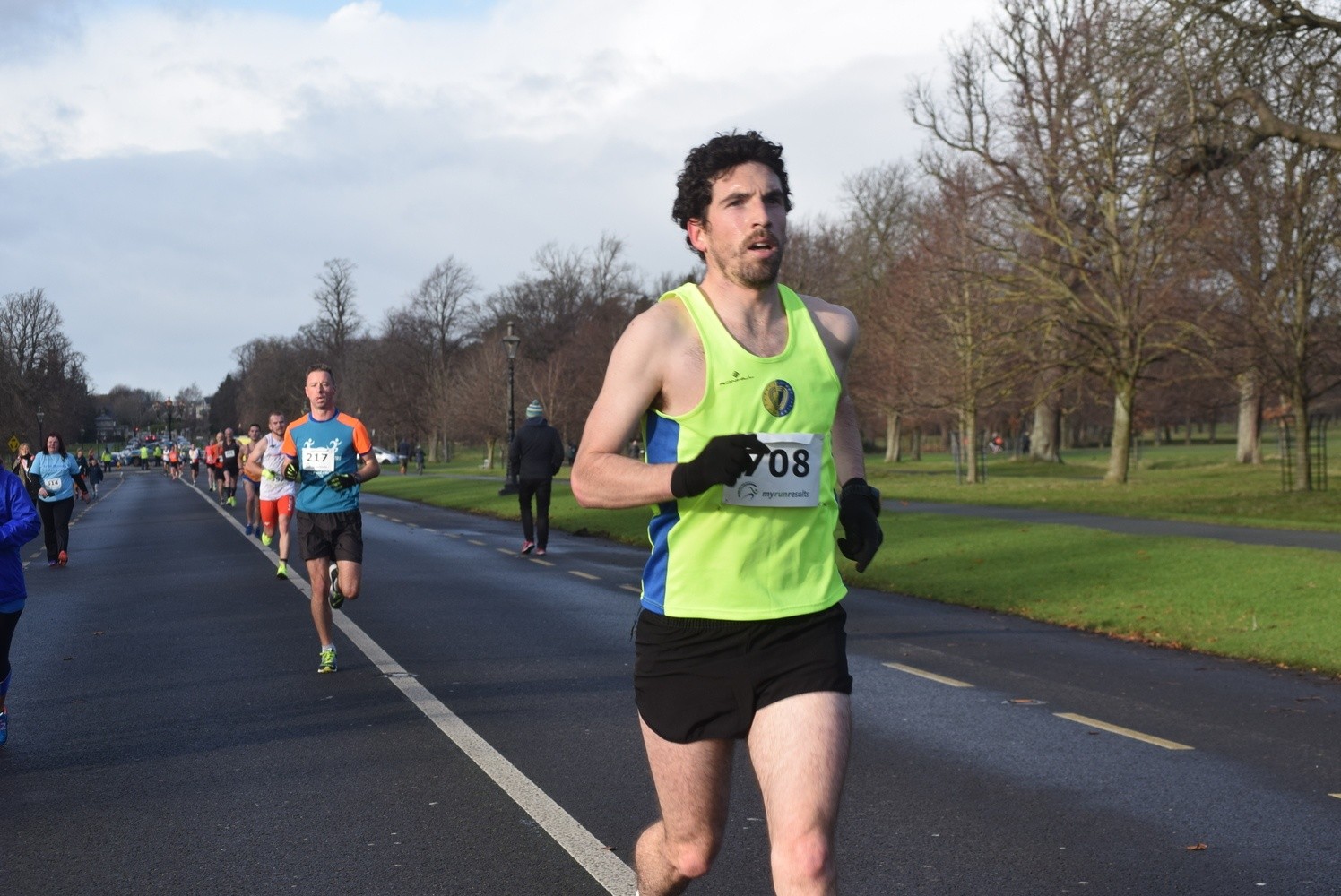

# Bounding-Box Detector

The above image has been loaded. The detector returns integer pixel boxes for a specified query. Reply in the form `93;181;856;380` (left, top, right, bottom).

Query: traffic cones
116;461;122;471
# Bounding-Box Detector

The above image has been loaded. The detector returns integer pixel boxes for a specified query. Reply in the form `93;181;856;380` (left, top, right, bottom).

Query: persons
0;364;427;748
506;400;577;556
988;429;1033;456
571;127;886;896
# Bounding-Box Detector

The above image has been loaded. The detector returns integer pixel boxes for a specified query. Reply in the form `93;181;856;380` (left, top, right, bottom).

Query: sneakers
0;705;8;748
328;564;344;610
522;540;535;554
318;647;337;672
262;531;272;546
536;548;545;555
277;561;288;577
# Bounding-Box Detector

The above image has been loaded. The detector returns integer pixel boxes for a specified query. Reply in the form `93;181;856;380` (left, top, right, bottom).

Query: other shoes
245;524;252;535
231;497;236;506
48;560;56;566
220;500;225;505
75;494;78;500
80;495;84;500
58;551;67;567
227;498;232;504
254;528;260;539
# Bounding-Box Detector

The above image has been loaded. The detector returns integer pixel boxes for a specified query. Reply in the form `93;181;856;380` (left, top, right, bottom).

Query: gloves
836;478;883;573
284;462;299;482
266;470;276;480
670;433;771;499
328;472;355;493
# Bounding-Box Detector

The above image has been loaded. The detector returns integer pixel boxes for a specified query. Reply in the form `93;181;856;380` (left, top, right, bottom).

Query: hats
526;399;543;418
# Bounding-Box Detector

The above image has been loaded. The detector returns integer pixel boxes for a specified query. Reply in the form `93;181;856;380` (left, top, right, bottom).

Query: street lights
35;406;45;452
80;425;85;450
155;395;186;442
498;322;521;495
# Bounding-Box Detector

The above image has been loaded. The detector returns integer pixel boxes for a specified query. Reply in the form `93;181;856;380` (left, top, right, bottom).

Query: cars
356;446;407;466
109;441;186;467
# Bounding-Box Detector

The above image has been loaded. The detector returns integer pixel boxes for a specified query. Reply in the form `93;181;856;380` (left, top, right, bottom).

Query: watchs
351;473;362;485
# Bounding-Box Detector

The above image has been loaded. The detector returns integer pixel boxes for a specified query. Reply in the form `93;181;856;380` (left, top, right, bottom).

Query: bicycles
414;453;426;475
